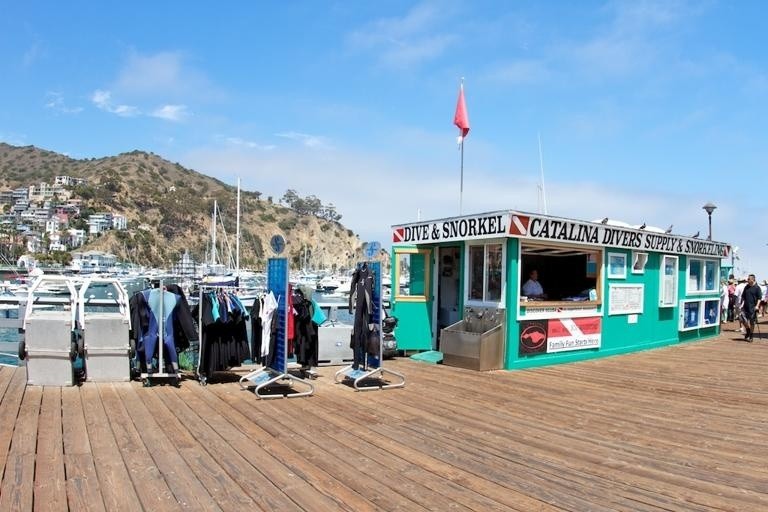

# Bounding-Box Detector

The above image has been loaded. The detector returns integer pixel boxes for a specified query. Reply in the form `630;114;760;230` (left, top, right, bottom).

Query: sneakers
734;328;753;343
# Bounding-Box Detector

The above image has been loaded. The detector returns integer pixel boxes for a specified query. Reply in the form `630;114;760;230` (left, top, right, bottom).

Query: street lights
702;200;717;240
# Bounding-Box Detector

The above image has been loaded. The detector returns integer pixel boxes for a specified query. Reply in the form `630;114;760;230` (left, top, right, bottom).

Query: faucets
467;311;483;322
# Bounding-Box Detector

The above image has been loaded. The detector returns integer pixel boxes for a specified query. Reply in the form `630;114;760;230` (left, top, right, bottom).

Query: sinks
439;317;505;371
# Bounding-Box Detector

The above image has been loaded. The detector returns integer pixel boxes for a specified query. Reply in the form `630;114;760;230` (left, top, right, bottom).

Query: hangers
204;284;238;295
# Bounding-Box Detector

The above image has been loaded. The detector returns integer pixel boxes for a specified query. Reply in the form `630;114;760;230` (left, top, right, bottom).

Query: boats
0;253;141;318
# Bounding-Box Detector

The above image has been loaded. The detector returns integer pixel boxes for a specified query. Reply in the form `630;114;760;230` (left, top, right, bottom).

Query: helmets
382;317;397;334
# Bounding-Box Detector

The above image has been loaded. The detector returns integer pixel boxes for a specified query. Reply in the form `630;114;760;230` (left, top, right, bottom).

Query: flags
453;81;470;148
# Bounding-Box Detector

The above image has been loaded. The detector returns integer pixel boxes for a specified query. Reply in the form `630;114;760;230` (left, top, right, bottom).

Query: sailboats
117;177;410;310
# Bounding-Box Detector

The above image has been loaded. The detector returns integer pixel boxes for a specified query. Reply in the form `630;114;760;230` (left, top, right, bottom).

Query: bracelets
540;294;542;297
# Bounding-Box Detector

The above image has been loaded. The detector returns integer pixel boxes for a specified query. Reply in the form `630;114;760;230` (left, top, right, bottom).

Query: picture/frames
607;252;627;280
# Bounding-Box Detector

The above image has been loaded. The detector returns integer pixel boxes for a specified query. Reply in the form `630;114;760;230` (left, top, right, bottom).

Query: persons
738;274;762;342
521;266;546;302
720;274;768;332
